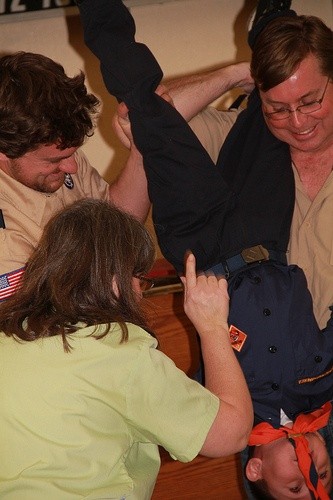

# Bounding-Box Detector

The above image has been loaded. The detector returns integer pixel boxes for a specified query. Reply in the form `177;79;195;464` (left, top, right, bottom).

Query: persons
0;51;150;313
69;0;333;500
0;200;254;500
164;15;333;329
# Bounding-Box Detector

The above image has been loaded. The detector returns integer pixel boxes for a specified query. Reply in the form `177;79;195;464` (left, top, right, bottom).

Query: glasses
132;273;155;290
261;72;331;120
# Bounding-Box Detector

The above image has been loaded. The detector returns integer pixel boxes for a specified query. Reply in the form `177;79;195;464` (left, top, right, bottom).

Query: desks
140;275;251;500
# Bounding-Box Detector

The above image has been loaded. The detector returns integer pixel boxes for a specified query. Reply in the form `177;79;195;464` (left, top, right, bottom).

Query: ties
247;400;333;500
